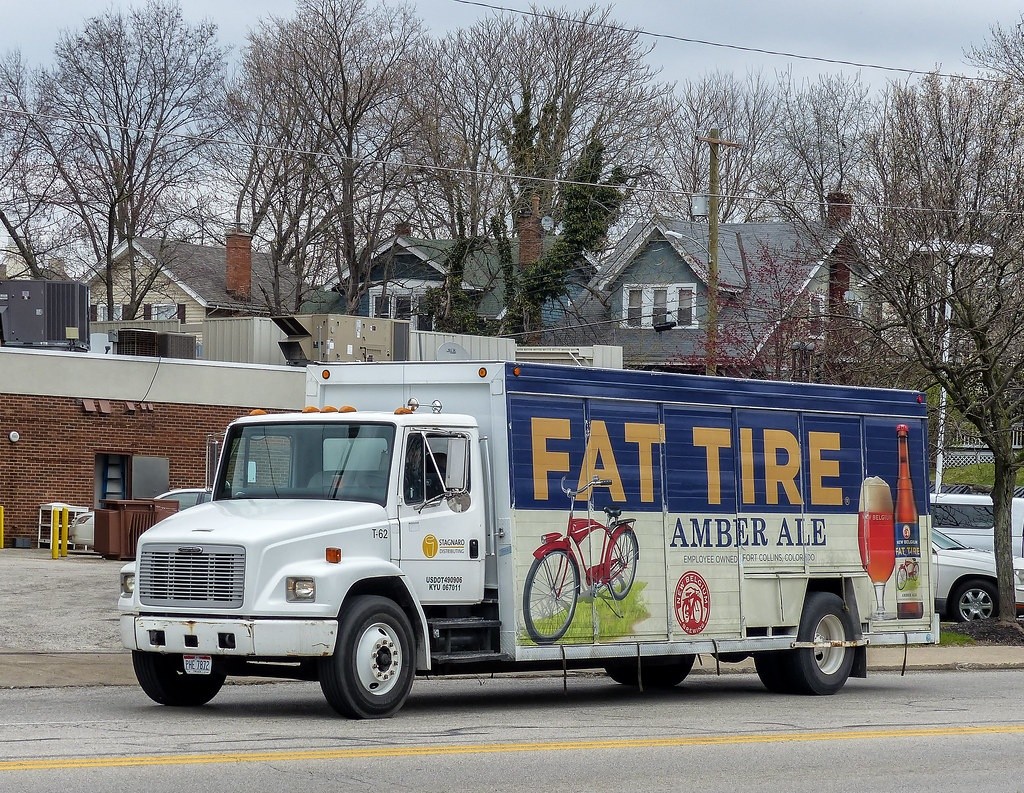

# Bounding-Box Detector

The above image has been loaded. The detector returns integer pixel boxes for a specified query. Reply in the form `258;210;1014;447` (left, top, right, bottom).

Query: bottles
895;424;923;619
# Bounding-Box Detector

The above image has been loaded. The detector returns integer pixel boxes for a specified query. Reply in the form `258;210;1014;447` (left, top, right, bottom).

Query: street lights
664;230;718;377
907;241;995;494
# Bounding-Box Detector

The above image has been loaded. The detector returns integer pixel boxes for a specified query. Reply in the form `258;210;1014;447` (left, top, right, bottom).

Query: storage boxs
12;538;31;548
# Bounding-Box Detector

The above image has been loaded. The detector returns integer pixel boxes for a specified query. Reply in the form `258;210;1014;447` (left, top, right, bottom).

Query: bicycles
522;475;640;644
897;558;919;590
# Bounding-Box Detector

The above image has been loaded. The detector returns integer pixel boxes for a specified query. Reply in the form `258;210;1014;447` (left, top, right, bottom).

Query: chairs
418;452;448;500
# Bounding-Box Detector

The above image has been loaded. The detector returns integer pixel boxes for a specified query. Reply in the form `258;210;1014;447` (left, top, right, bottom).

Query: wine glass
858;484;898;620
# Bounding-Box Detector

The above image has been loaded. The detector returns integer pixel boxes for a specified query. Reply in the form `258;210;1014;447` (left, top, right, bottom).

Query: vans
929;493;1024;560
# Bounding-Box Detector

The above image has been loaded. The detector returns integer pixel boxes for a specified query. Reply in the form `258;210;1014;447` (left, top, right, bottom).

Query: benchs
307;469;387;489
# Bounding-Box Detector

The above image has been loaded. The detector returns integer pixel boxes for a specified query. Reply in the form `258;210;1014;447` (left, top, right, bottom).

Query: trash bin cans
90;497;179;561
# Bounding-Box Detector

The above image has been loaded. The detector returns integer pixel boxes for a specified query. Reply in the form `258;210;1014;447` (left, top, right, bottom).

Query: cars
69;487;212;547
930;529;1024;625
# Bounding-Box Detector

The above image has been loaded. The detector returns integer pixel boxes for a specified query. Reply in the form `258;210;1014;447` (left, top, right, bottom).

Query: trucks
117;358;942;720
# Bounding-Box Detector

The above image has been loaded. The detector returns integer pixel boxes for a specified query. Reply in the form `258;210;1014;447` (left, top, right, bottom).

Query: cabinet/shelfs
38;502;89;551
